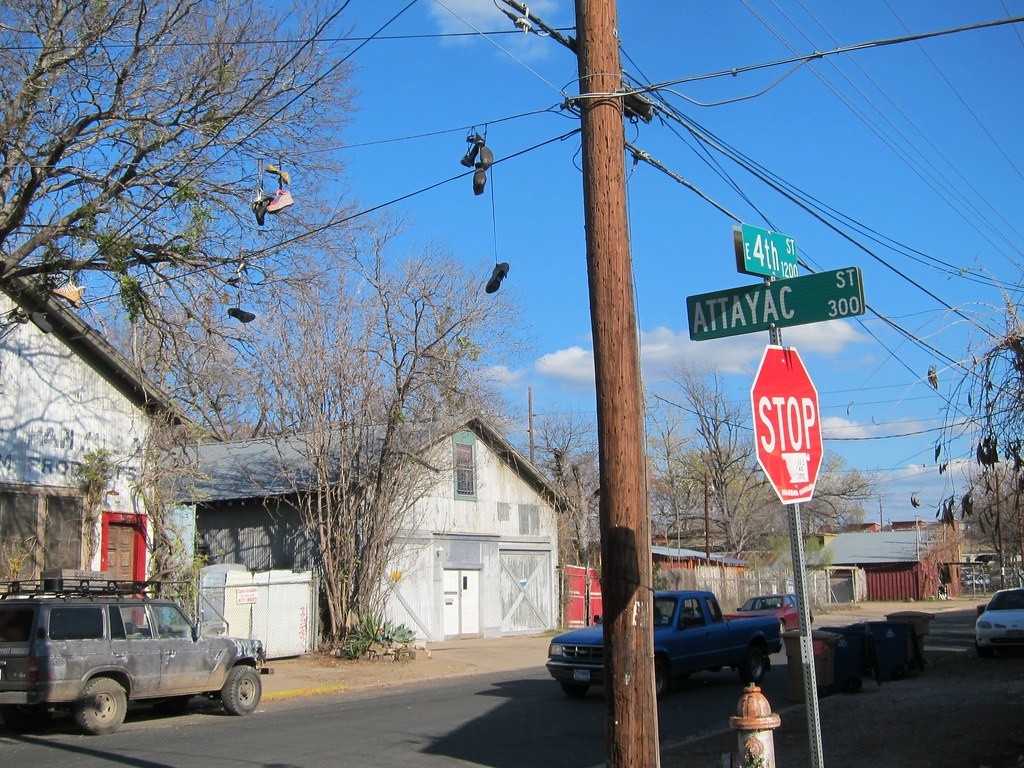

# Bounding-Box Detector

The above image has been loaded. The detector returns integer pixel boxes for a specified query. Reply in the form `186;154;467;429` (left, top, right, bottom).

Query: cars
975;588;1024;658
940;567;993;591
966;574;991;587
736;593;814;634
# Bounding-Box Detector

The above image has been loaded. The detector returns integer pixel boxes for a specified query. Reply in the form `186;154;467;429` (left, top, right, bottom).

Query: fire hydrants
721;682;781;768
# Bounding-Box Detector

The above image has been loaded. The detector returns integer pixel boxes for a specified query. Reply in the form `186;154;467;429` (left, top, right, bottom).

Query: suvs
0;579;275;735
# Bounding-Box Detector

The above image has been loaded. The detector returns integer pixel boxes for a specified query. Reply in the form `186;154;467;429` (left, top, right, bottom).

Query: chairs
125;623;142;638
678;607;694;625
159;624;166;638
654;608;661;625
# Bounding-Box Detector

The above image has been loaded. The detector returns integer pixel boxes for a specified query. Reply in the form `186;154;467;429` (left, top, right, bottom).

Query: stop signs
750;345;823;505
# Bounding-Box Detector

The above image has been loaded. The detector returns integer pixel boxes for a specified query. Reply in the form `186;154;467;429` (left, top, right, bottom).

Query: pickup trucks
546;590;782;699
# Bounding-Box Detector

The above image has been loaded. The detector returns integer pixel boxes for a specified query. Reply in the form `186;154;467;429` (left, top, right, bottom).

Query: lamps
107;482;119;496
